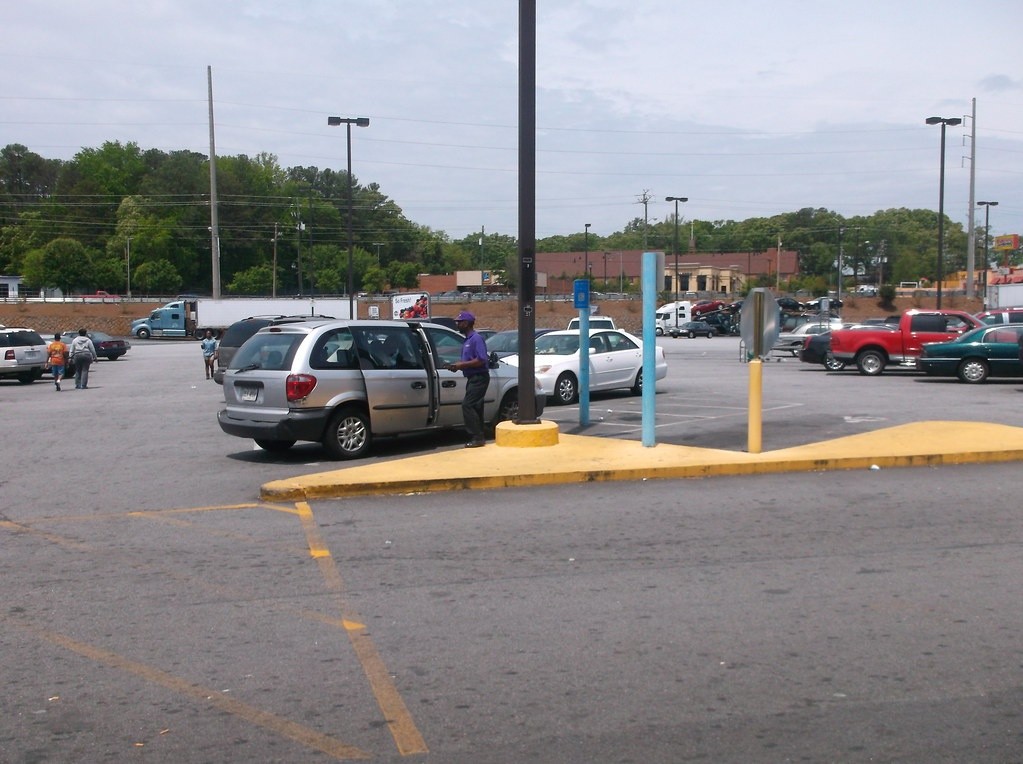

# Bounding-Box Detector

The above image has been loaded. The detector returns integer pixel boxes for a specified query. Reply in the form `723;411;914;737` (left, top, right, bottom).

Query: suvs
213;315;336;384
0;326;48;384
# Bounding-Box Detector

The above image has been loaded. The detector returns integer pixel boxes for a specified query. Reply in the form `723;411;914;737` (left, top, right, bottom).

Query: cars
948;306;1023;332
39;331;131;379
217;316;667;461
727;285;900;358
916;322;1023;385
691;300;726;316
669;321;717;339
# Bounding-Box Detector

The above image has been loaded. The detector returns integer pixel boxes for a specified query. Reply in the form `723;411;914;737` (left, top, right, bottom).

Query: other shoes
466;439;484;447
56;380;61;389
58;387;61;391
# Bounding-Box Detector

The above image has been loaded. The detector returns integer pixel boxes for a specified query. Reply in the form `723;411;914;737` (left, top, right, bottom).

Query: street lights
589;263;592;291
926;117;962;310
977;201;999;312
585;224;591;277
127;237;135;297
665;197;688;327
327;116;370;319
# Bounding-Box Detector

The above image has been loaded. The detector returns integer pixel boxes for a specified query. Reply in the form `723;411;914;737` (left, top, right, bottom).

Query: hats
454;311;475;323
54;333;61;339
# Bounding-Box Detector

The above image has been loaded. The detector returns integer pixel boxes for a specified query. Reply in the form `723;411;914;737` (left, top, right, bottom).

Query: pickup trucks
77;291;121;297
830;309;1018;376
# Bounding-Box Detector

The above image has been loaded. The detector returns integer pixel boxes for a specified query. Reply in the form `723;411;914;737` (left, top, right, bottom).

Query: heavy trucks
656;302;840;337
131;297;358;340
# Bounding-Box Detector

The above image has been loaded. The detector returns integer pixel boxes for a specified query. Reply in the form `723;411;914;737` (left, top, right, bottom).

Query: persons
69;328;98;389
201;329;218;380
45;333;67;392
448;311;490;448
